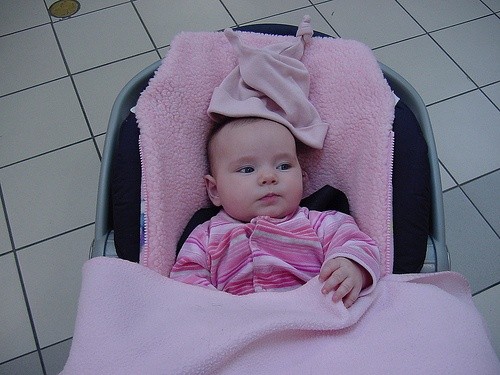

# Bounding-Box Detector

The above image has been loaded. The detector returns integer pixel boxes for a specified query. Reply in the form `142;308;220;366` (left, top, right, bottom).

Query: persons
168;116;384;310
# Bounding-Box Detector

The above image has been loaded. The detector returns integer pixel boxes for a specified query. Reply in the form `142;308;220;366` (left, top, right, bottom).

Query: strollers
60;23;500;375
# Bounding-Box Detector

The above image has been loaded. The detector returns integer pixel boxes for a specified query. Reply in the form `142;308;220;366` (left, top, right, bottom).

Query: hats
206;14;329;150
